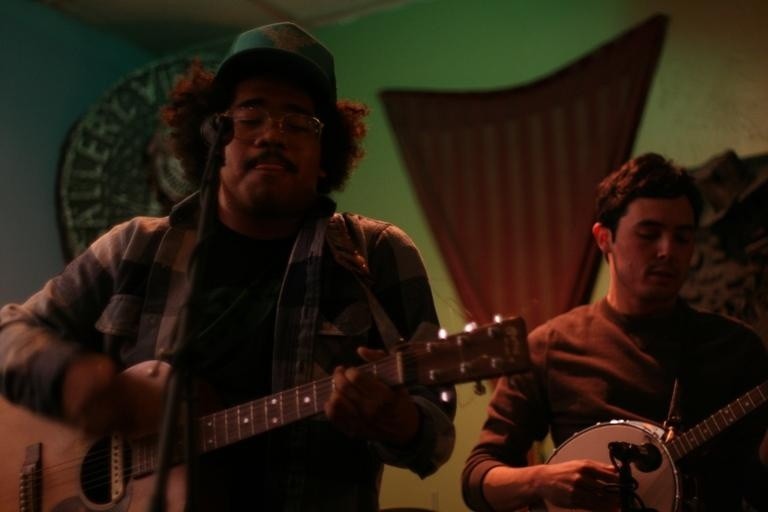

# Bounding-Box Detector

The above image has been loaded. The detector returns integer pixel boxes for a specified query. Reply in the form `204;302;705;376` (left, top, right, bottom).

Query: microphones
608;439;663;473
198;111;237;148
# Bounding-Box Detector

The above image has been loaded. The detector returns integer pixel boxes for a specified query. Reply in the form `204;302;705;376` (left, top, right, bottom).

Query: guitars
0;314;533;512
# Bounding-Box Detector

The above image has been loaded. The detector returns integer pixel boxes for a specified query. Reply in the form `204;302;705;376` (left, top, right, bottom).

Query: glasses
222;105;323;149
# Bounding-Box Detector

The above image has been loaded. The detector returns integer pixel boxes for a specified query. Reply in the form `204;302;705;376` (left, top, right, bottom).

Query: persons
0;22;457;510
460;152;768;512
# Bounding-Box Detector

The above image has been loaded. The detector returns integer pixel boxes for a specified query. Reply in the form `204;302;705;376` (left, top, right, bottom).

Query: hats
697;149;767;229
215;20;337;100
